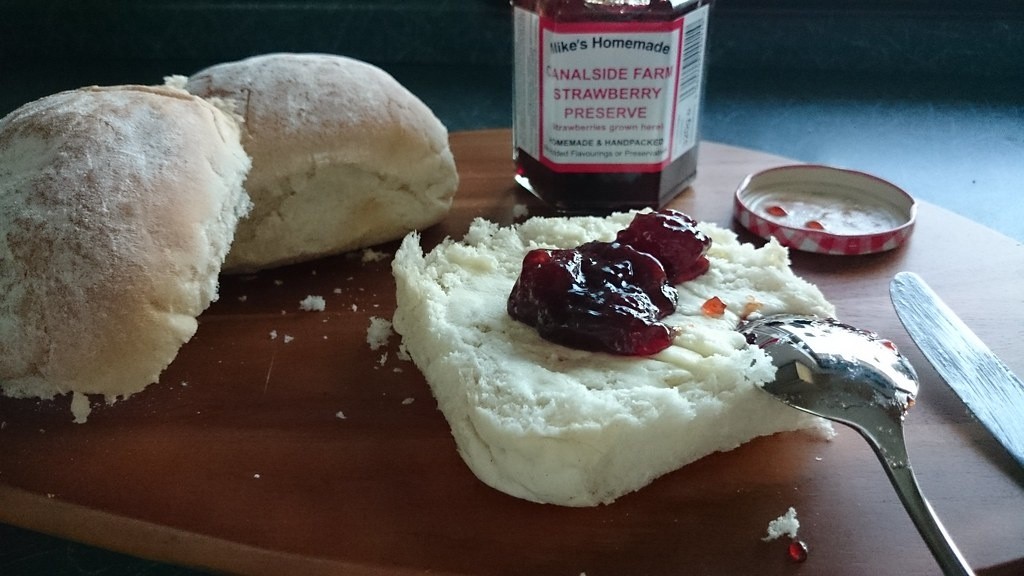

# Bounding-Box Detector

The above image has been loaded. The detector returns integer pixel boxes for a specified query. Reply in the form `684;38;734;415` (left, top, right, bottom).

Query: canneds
506;0;710;213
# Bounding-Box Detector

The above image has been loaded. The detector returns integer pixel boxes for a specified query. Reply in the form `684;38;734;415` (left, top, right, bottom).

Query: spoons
731;307;983;576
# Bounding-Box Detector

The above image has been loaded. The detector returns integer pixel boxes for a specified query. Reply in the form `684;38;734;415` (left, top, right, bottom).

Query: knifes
888;269;1024;463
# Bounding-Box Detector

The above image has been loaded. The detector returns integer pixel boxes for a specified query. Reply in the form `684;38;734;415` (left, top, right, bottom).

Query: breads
0;80;253;422
155;53;460;271
384;203;845;507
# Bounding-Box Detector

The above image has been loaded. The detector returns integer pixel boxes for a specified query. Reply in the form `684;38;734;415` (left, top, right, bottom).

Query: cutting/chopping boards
0;121;1024;576
